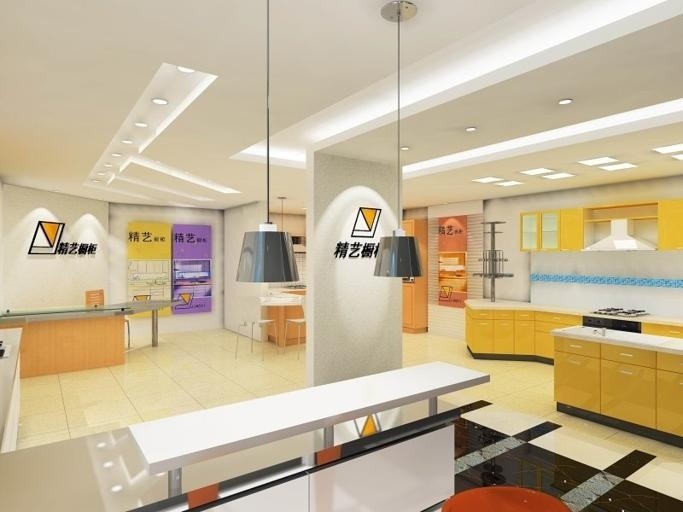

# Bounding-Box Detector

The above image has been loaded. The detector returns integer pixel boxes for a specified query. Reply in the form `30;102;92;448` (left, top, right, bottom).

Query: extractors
580;218;659;253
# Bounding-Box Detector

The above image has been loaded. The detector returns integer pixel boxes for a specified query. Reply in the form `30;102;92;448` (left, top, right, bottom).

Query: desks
128;361;490;497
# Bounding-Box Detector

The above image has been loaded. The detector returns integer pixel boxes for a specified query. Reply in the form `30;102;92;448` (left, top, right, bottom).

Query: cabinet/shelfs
464;309;580;364
520;198;683;252
553;337;683;448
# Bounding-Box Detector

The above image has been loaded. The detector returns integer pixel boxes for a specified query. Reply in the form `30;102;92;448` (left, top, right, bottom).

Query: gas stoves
591;305;651;319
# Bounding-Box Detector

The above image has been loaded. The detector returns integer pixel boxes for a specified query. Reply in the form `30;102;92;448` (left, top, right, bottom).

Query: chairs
283;295;305;360
234;295;279;363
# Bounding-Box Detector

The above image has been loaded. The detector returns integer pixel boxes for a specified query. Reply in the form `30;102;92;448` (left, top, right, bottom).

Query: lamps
236;0;299;282
375;0;423;277
